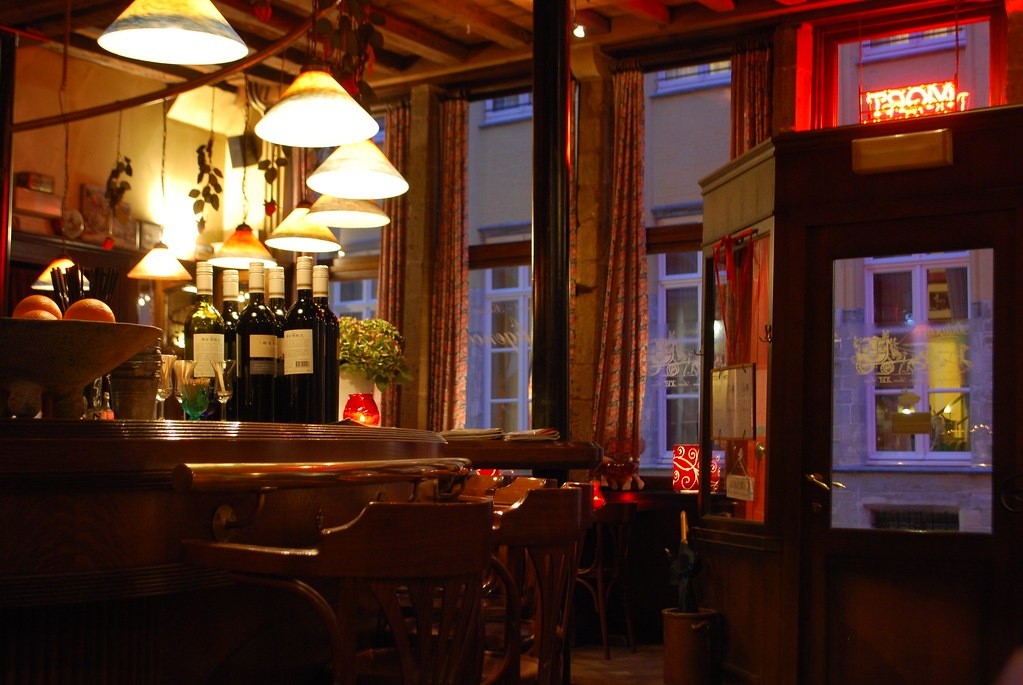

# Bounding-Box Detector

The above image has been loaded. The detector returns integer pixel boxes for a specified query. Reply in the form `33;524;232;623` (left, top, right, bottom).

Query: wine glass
181;378;210;419
155;355;178;420
210;361;235;420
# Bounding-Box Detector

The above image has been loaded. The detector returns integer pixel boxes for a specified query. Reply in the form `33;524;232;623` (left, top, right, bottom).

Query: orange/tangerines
12;295;62;320
20;310;58;320
62;298;115;323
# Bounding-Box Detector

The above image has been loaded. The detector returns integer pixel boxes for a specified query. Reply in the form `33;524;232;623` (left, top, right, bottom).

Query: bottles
220;270;241;421
269;266;287;423
235;261;277;423
281;256;325;425
343;393;380;426
184;261;225;420
311;265;340;425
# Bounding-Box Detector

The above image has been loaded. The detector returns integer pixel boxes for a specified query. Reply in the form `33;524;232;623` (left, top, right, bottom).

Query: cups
110;338;162;419
173;360;197;403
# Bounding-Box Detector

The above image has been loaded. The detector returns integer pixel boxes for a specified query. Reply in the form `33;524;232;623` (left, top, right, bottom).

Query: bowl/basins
0;318;164;419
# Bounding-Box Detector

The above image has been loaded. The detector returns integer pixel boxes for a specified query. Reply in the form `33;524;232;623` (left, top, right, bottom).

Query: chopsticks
50;264;120;317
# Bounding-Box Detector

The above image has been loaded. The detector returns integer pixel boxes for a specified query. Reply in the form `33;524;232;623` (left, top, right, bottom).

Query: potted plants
338;316;413;428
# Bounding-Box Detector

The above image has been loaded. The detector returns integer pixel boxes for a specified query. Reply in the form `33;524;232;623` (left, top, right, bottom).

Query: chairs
183;474;638;685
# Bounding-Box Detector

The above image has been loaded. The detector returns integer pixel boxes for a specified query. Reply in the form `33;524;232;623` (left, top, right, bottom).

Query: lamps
672;444;699;495
30;0;409;290
